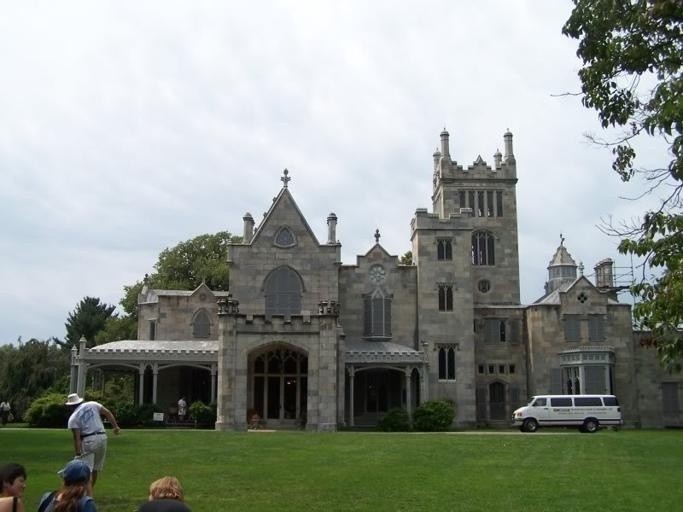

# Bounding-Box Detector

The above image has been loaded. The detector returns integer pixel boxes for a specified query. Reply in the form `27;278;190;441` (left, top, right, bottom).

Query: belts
84;431;105;437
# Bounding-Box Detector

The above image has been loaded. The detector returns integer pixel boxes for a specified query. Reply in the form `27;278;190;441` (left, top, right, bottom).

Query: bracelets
75;452;81;455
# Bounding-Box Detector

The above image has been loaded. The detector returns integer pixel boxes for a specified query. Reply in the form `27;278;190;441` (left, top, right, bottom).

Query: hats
58;460;90;481
64;393;83;406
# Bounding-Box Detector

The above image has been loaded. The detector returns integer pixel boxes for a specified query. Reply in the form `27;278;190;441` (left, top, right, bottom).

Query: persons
0;399;12;427
0;462;27;511
64;392;120;496
35;459;96;511
135;475;191;511
177;394;187;423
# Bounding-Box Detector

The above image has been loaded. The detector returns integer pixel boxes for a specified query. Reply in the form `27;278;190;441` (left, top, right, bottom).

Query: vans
511;394;623;433
